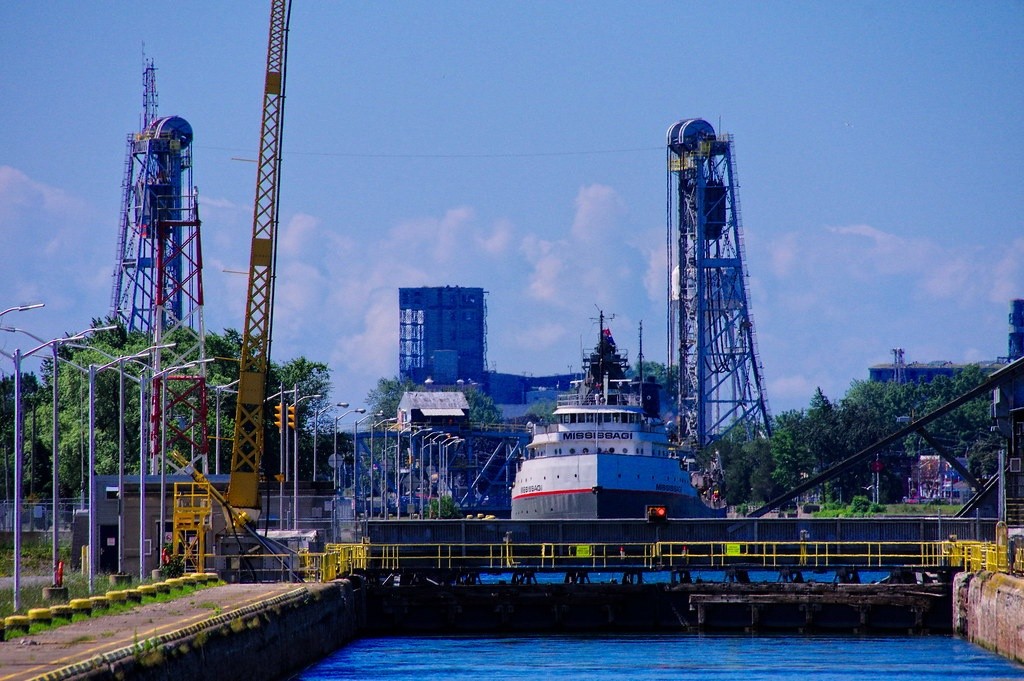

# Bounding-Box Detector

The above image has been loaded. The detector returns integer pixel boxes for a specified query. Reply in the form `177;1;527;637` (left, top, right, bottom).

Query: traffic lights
274;403;282;433
288;407;296;430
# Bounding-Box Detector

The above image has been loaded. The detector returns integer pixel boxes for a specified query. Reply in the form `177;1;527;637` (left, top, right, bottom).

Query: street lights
334;408;366;489
385;422;465;533
293;384;322;529
313;403;349;481
371;418;399;520
354;413;383;491
0;303;240;616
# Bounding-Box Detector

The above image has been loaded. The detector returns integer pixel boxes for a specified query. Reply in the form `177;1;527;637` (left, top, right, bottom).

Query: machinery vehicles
214;0;310;585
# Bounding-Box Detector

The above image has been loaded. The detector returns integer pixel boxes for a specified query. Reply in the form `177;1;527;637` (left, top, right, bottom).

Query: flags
603;328;616;345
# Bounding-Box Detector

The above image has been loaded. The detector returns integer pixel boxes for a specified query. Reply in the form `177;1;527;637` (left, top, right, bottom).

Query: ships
509;305;728;518
